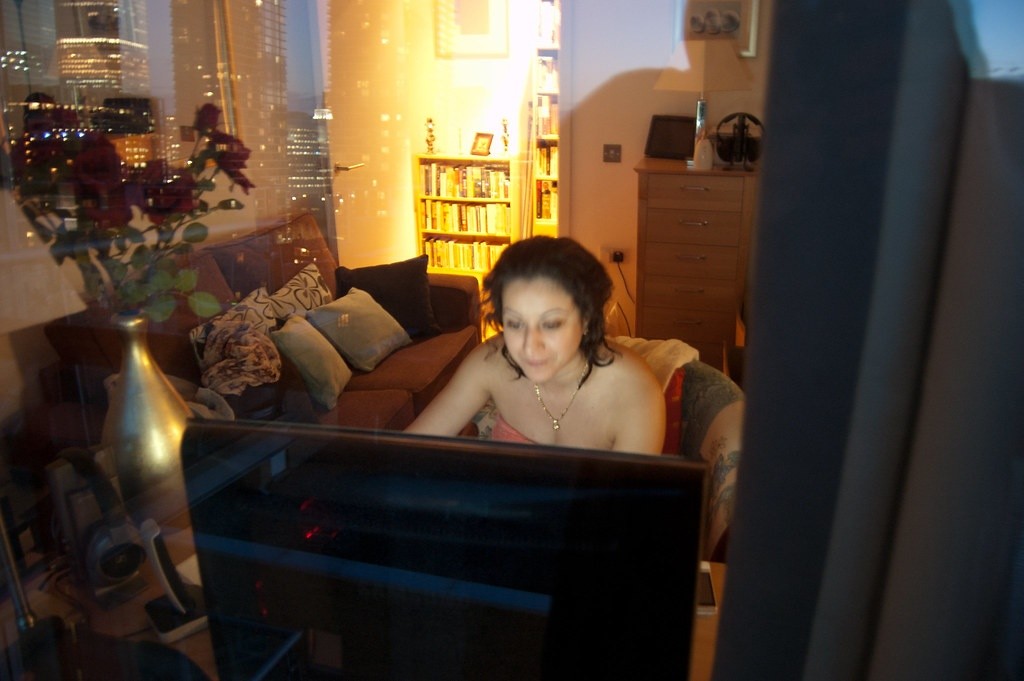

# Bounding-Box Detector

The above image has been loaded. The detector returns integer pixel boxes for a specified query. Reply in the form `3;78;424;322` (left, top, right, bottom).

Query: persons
402;235;666;455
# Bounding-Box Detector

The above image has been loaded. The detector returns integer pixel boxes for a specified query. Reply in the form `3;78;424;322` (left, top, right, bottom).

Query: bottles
694;137;713;170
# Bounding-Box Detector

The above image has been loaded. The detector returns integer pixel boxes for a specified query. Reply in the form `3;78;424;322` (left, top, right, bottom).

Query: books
536;95;559;218
420;163;509;271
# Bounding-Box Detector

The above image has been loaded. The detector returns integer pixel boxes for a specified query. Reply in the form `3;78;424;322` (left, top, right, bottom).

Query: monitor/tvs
176;416;708;681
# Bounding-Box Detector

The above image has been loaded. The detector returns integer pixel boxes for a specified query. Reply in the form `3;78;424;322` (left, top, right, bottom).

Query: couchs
18;210;500;531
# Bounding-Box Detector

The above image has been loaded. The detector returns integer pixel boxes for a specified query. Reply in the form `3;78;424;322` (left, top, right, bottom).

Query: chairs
461;337;745;560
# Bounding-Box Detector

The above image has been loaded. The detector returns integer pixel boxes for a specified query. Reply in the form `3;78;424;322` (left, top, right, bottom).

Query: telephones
139;518;208;645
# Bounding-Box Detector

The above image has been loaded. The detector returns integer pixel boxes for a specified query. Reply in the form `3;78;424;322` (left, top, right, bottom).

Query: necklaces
533;363;588;430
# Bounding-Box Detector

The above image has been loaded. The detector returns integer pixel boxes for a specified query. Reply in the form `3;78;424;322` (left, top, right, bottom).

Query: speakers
140;520;210;642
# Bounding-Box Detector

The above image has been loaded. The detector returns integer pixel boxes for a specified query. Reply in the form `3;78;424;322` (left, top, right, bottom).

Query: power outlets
606;246;630;264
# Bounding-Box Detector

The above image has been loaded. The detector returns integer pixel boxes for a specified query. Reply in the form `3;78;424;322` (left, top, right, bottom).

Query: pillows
271;312;355;411
307;286;412;371
272;261;333;316
336;252;441;337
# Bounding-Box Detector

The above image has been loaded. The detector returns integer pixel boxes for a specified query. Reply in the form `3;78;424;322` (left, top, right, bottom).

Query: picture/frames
432;0;510;59
470;132;495;155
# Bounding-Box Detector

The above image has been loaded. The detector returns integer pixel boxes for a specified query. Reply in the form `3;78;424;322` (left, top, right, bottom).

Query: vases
95;306;203;537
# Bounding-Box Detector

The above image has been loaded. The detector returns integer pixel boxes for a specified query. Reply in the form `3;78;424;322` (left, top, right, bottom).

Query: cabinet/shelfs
531;5;560;237
631;156;751;371
408;154;518;285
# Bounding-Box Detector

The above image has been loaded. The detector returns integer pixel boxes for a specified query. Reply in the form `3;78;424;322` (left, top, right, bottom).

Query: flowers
13;92;254;321
